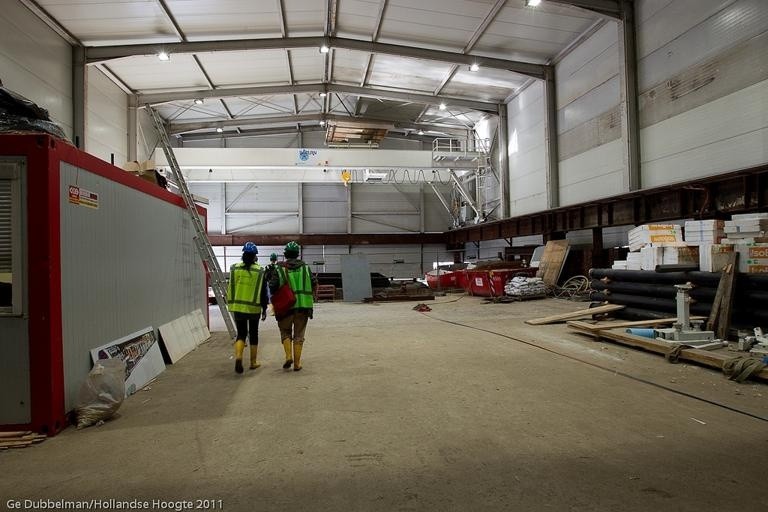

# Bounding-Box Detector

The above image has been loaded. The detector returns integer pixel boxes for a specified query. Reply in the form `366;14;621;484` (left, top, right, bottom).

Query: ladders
146;103;237;328
192;235;237;340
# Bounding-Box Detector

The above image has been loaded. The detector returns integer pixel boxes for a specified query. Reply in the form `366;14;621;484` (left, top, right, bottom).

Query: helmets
242;242;259;255
283;240;300;254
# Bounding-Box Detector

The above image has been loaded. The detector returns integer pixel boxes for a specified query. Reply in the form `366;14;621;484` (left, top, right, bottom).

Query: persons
269;242;319;372
227;240;268;374
265;252;278;316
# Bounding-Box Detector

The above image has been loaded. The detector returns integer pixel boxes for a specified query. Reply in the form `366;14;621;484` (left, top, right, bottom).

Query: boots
282;337;293;369
234;339;244;374
293;341;303;371
249;345;262;369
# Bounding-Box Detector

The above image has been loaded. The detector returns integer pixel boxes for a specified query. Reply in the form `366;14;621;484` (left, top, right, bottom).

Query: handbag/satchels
269;282;296;315
